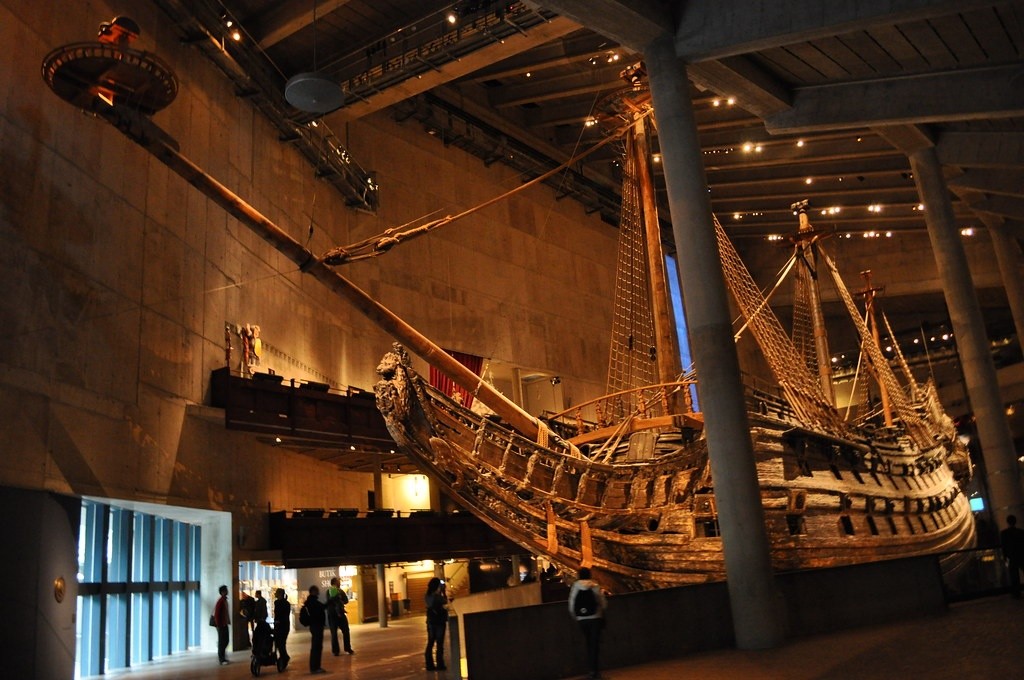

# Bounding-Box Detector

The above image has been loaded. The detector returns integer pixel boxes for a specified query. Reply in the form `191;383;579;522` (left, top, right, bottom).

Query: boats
39;0;978;591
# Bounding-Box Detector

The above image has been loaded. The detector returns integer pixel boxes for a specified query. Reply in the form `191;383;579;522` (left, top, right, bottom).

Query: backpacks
300;605;311;627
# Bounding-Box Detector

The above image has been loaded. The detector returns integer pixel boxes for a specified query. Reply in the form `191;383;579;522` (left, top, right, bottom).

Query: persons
326;578;353;656
999;516;1024;601
240;590;275;667
213;585;231;666
274;588;290;672
304;585;326;673
424;578;447;671
568;567;609;680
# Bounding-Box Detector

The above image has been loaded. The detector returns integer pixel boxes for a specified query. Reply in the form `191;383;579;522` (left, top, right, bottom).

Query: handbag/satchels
209;615;217;626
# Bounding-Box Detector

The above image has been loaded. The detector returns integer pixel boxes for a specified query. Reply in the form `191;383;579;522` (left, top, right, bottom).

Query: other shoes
217;660;230;665
311;668;327;674
426;665;437;671
436;666;447;670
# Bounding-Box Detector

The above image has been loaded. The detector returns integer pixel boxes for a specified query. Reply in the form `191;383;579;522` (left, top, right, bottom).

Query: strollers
250;622;286;676
